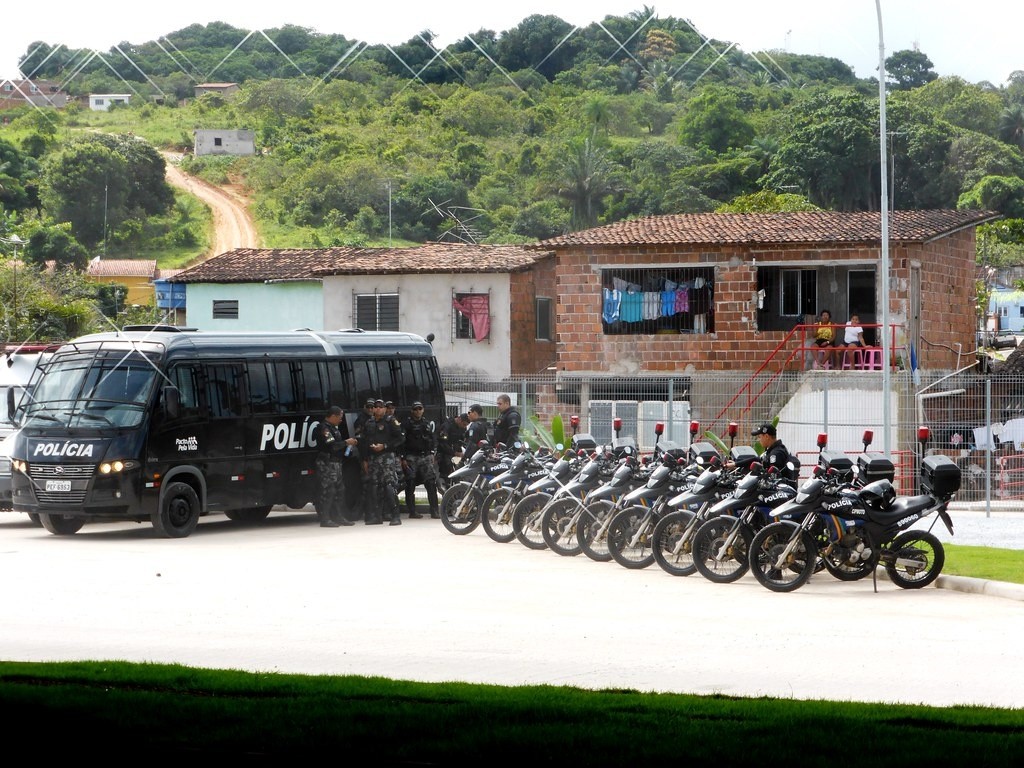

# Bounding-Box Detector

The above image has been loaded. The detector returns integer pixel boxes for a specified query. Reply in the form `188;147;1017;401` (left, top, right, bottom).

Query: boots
406;494;423;518
428;492;440;518
320;501;355;527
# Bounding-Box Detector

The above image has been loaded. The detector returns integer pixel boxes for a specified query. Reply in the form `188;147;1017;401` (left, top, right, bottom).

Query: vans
1;324;444;538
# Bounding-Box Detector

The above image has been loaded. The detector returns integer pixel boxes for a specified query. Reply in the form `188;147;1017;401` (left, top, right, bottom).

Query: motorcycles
439;416;962;593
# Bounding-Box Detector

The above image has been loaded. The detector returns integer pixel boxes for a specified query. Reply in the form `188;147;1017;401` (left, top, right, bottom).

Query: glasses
366;404;374;409
413;407;422;410
374;404;384;408
468;411;471;414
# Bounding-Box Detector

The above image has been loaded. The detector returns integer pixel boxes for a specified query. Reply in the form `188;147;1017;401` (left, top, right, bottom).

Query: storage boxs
919;453;962;499
730;445;759;462
690;441;720;468
819;450;854;482
571;433;596;456
656;440;686;466
857;451;895;485
789;456;801;480
613;436;637;458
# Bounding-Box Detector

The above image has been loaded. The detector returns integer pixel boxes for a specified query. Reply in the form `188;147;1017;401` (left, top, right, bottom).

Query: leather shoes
365;517;383;525
384;514;401;525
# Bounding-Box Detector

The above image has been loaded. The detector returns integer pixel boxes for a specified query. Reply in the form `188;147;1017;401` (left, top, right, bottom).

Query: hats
366;398;385;406
412;401;424;409
385;401;397;407
751;424;777;436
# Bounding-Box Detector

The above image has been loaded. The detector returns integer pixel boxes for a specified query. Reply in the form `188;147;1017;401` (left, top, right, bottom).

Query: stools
811;347;834;370
888;346;905;371
842;349;863;370
862;349;883;370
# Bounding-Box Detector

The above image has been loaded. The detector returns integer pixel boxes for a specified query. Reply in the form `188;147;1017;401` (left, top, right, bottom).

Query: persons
400;401;440;518
723;424;791;580
449;404;487;523
810;310;836;369
832;315;873;370
316;406;355;527
437;413;471;516
354;398;404;526
493;395;521;508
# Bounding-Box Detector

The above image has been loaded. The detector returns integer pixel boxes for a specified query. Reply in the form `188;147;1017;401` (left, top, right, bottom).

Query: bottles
344;445;352;456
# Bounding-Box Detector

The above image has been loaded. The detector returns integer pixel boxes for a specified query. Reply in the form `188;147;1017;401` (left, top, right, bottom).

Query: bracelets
827;341;830;344
